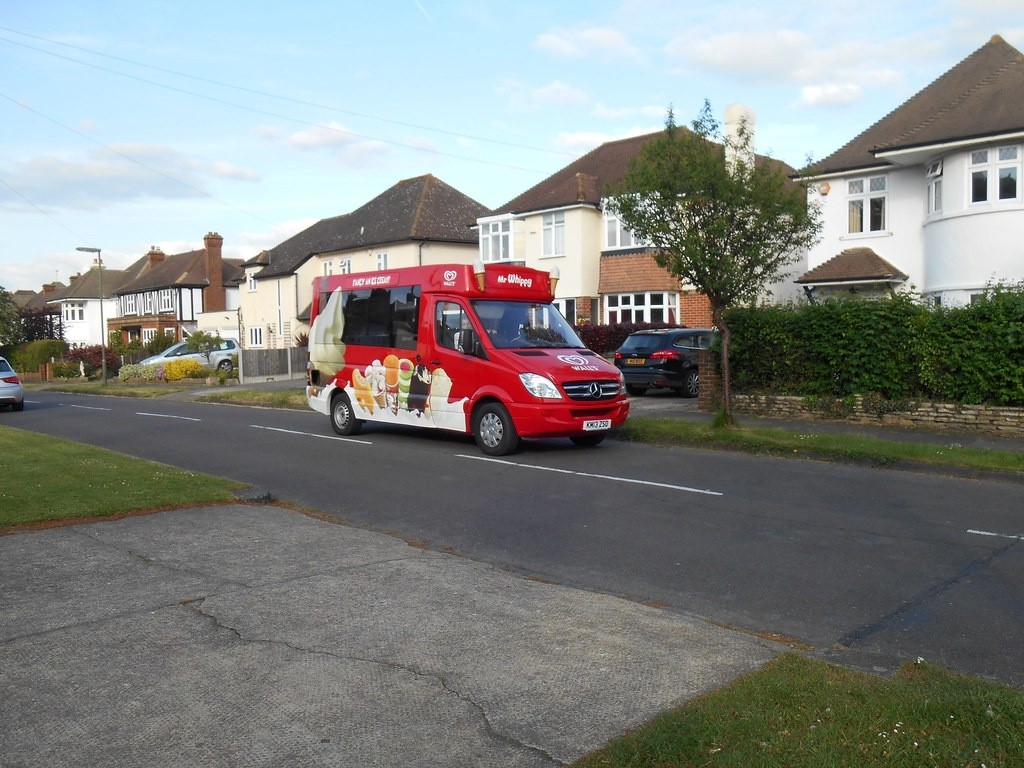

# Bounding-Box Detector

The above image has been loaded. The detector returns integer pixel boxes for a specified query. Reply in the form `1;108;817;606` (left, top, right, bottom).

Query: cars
0;357;24;412
138;338;241;374
613;328;716;399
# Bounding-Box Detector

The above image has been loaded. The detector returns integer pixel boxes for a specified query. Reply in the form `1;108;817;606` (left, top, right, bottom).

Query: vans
305;263;631;456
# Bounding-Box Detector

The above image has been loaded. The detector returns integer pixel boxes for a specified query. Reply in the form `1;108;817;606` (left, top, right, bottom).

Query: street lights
76;247;108;386
